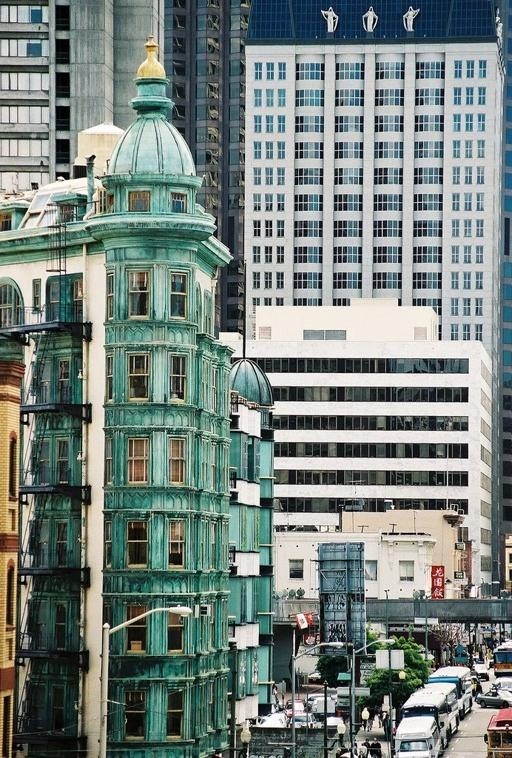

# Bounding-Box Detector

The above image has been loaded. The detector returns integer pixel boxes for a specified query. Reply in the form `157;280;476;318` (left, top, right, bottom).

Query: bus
394;638;512;758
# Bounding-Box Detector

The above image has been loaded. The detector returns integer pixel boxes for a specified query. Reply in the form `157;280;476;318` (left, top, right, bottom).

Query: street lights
478;581;500;599
292;629;344;758
96;606;193;758
351;639;396;750
424;588;462;663
384;588;388;639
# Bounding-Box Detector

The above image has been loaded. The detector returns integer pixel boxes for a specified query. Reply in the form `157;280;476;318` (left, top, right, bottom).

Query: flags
296;610;314;629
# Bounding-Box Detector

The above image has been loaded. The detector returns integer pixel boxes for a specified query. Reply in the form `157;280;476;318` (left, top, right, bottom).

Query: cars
255;693;351;731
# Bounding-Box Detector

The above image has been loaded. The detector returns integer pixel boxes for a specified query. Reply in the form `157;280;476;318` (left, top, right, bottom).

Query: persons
336;705;391;758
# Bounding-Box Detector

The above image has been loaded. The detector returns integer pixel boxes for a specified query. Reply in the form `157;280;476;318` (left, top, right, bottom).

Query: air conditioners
201;604;213;619
